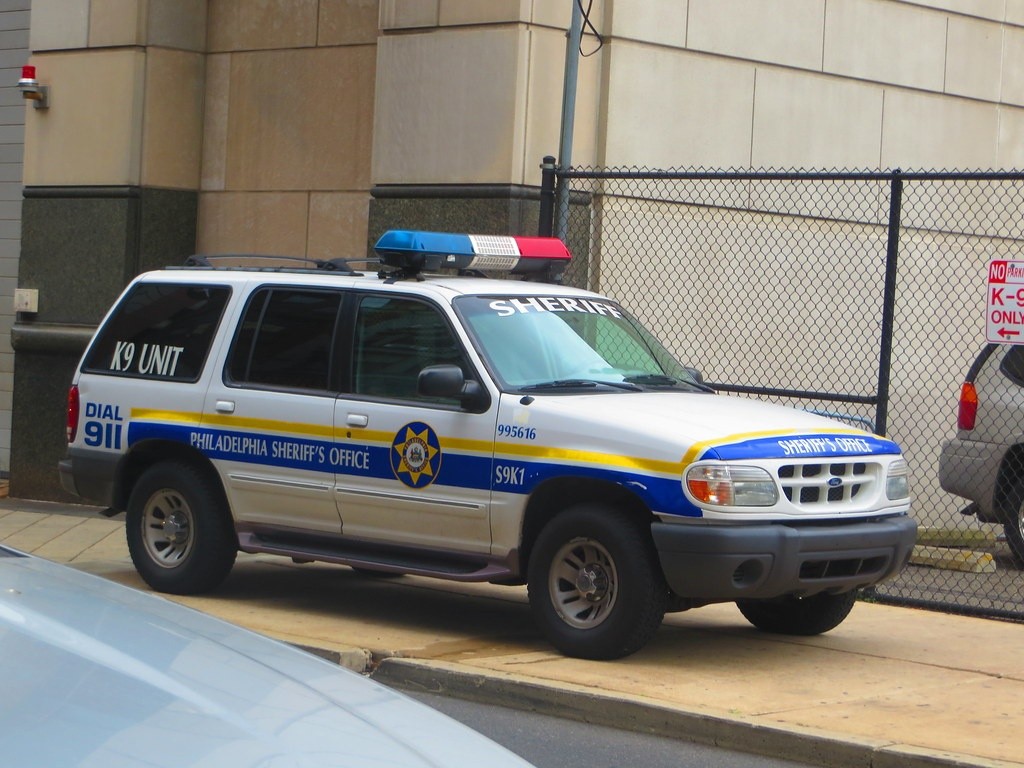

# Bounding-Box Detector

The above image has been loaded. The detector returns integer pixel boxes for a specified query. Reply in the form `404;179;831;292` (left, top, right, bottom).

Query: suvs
937;343;1024;567
55;228;919;661
